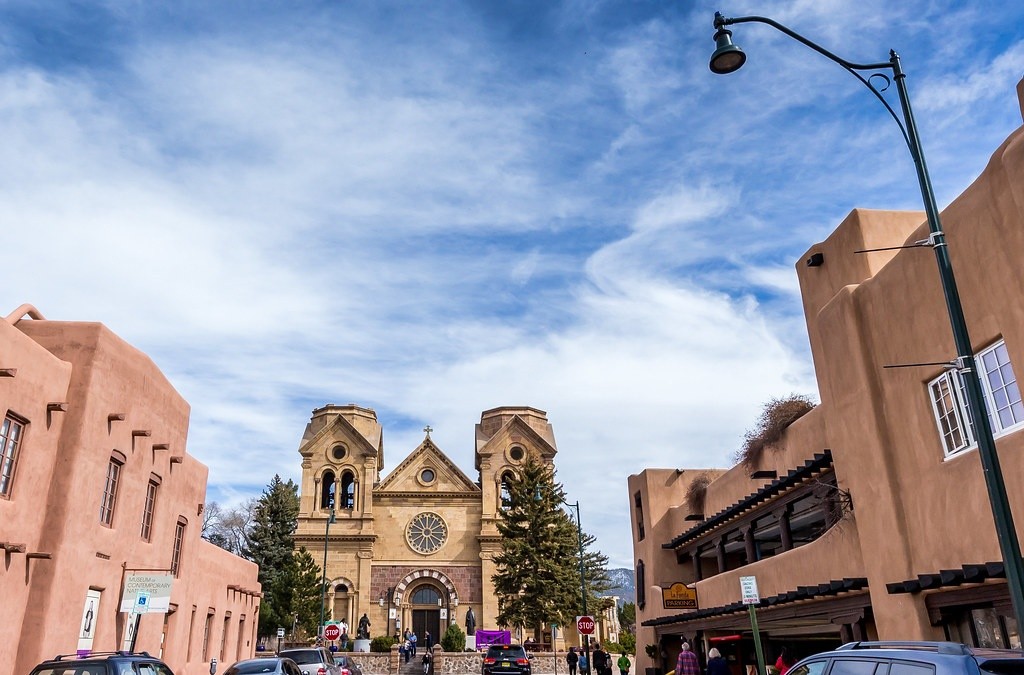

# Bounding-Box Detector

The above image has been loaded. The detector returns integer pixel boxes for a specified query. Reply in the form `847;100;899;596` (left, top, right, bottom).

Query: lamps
396;614;400;629
451;615;455;625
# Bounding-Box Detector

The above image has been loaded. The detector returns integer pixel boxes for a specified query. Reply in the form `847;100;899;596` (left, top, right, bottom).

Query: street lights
438;587;458;628
534;483;591;675
320;510;338;635
379;587;400;636
709;11;1024;650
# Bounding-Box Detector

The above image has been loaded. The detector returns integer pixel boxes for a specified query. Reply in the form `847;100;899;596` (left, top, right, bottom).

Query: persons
311;641;316;647
404;628;417;662
359;613;370;638
618;652;630;675
466;606;476;635
524;637;538;652
677;643;700;675
579;651;587;675
707;648;732;675
83;601;94;638
129;613;135;639
775;646;798;675
593;644;612;675
424;630;433;653
340;631;348;649
567;647;578;675
422;651;429;675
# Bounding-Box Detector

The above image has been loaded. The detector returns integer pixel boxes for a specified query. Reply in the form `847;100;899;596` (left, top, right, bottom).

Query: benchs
524;643;550;653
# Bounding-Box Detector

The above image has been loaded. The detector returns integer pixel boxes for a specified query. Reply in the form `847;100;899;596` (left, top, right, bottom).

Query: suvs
29;650;175;675
783;641;1023;675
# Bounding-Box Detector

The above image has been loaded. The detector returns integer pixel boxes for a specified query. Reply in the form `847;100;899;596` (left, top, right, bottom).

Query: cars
279;647;343;675
332;656;362;675
223;657;311;675
480;644;533;675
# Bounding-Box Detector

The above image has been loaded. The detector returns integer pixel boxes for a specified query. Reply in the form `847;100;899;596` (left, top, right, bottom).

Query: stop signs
576;616;595;635
324;624;340;641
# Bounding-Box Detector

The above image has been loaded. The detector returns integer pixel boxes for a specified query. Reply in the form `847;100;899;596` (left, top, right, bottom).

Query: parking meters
210;659;217;675
